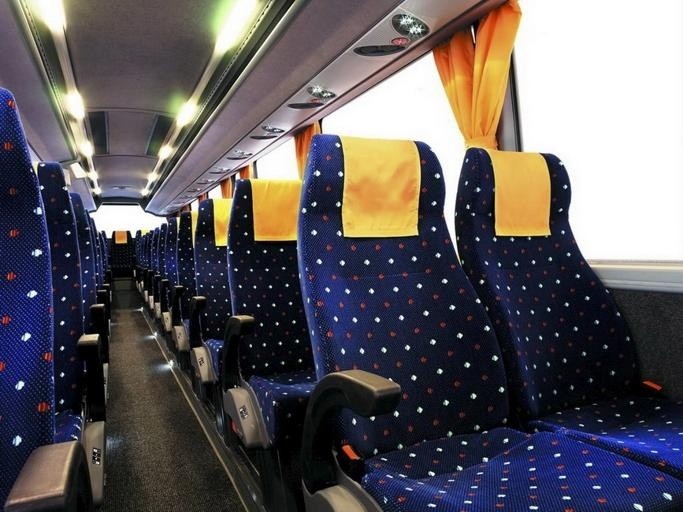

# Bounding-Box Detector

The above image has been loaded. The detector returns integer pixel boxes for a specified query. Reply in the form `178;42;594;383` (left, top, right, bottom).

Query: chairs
110;230;134;265
